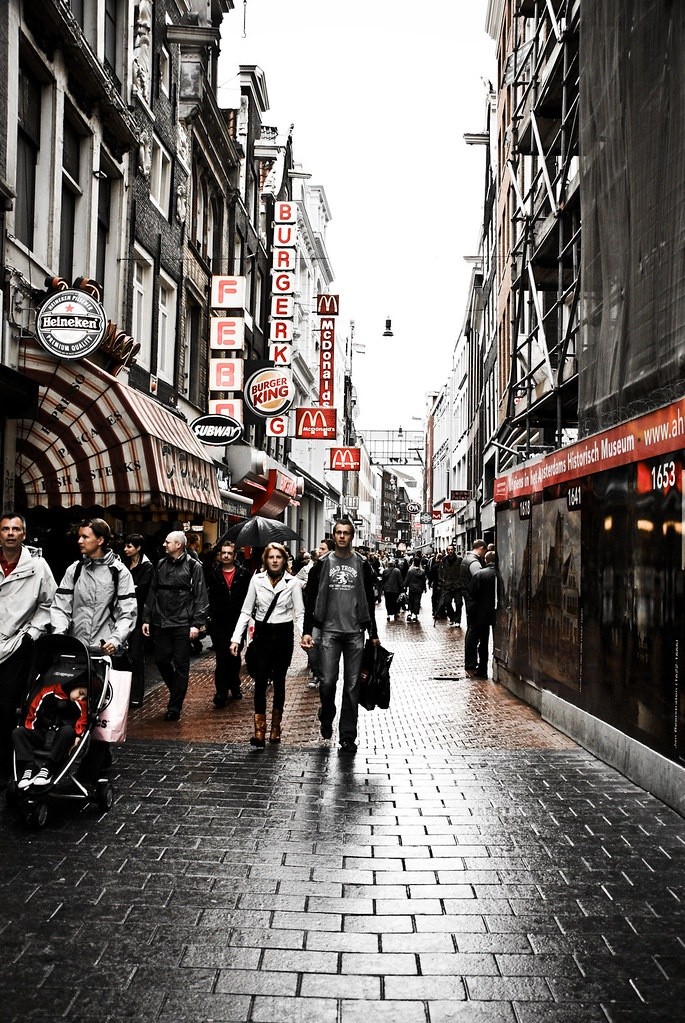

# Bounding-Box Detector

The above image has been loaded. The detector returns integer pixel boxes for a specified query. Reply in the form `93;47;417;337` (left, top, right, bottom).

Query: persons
0;512;138;789
124;518;380;753
186;533;496;710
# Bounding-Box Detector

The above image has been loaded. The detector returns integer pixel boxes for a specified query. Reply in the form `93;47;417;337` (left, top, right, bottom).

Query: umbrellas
210;515;305;574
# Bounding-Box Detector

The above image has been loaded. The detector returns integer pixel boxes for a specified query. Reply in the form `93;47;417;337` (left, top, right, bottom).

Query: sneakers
18;762;56;788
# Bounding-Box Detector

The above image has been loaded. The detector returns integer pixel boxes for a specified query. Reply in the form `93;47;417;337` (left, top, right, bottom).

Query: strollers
7;632;114;830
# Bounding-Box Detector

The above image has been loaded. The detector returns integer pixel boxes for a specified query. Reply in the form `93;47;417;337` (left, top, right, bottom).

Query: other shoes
308;676;320;688
407;617;417;623
213;688;242;703
449;621;460;628
465;668;487;680
340;738;357;751
129;697;144;705
387;615;399;622
165;710;180;721
320;721;332;739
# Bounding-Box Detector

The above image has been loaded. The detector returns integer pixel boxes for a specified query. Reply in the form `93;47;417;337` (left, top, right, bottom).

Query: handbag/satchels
92;655;133;743
357;638;394;711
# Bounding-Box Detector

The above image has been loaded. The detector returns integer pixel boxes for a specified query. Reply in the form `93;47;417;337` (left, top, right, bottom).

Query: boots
269;709;284;743
250;713;266;748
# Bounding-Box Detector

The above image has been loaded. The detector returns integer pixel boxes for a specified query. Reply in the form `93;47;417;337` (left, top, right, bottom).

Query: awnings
15;339;224;524
